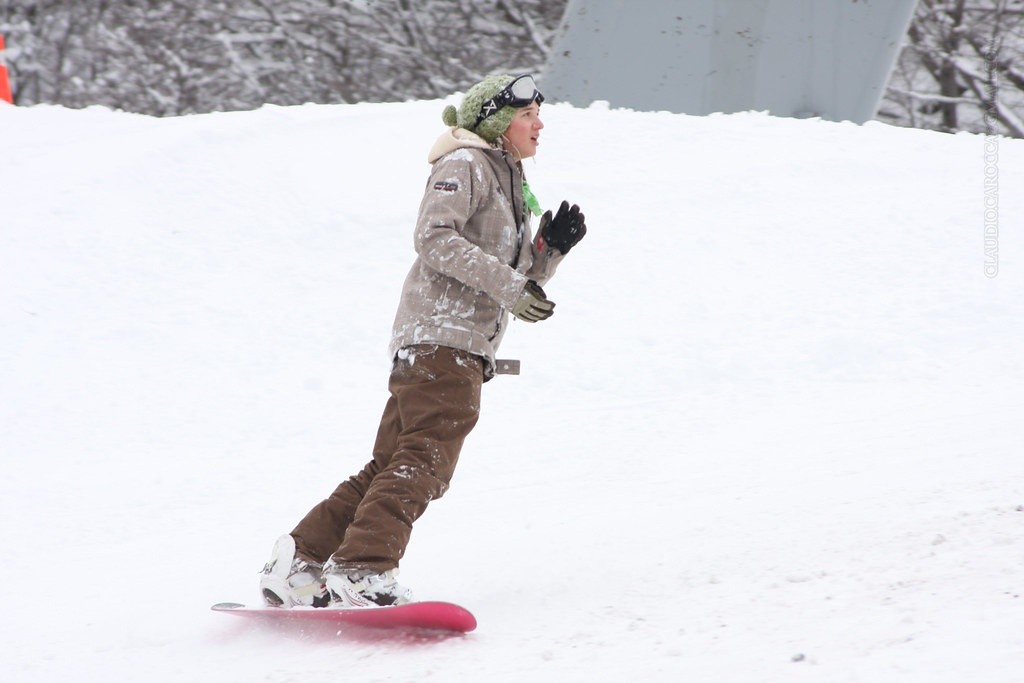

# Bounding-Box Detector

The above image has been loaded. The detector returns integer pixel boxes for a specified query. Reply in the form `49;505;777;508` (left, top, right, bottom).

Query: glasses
469;74;544;135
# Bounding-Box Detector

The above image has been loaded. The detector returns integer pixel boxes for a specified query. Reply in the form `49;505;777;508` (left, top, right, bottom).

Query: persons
261;75;587;607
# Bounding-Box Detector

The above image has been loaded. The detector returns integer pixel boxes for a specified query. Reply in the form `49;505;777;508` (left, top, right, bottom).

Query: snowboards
210;599;479;643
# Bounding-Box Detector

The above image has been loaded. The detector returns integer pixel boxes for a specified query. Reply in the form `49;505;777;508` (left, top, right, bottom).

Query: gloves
526;199;587;287
511;279;556;323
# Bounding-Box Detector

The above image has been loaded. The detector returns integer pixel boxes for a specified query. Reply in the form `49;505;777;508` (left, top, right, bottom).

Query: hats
441;74;520;142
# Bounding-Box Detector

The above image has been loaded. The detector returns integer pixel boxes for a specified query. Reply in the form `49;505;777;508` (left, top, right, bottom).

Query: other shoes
315;556;414;608
260;533;330;606
